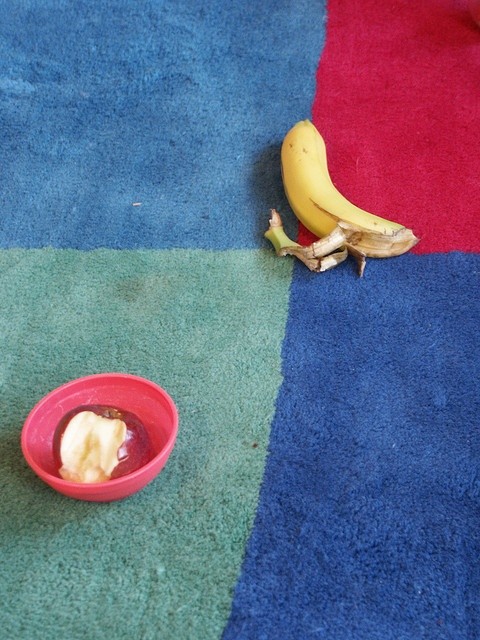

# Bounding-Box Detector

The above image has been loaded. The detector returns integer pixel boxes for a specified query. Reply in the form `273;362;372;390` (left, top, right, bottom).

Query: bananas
265;119;420;279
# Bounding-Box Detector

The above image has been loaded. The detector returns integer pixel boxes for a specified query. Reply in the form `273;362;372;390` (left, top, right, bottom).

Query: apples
52;405;128;484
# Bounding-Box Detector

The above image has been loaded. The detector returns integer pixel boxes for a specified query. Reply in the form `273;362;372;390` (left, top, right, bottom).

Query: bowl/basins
17;370;182;506
